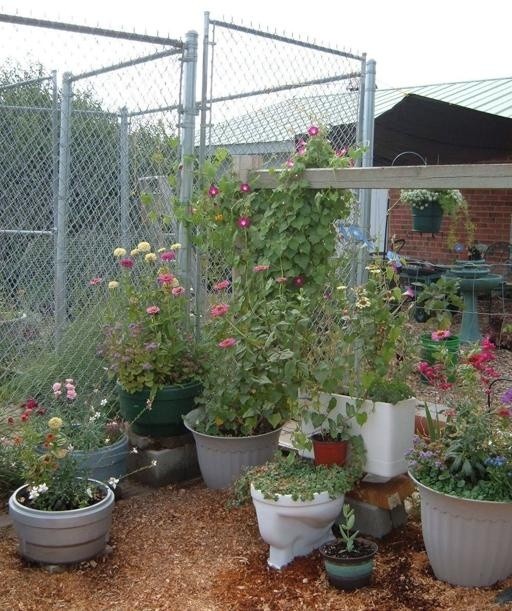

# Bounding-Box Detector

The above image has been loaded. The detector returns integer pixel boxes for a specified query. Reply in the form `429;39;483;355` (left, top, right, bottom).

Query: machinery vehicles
367;239;460;323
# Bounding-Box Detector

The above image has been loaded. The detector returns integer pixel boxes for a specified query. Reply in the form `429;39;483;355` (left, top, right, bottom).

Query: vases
408;460;512;588
6;477;115;569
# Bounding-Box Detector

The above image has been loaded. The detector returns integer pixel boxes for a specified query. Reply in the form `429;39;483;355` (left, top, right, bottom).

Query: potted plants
318;503;380;590
225;439;364;570
